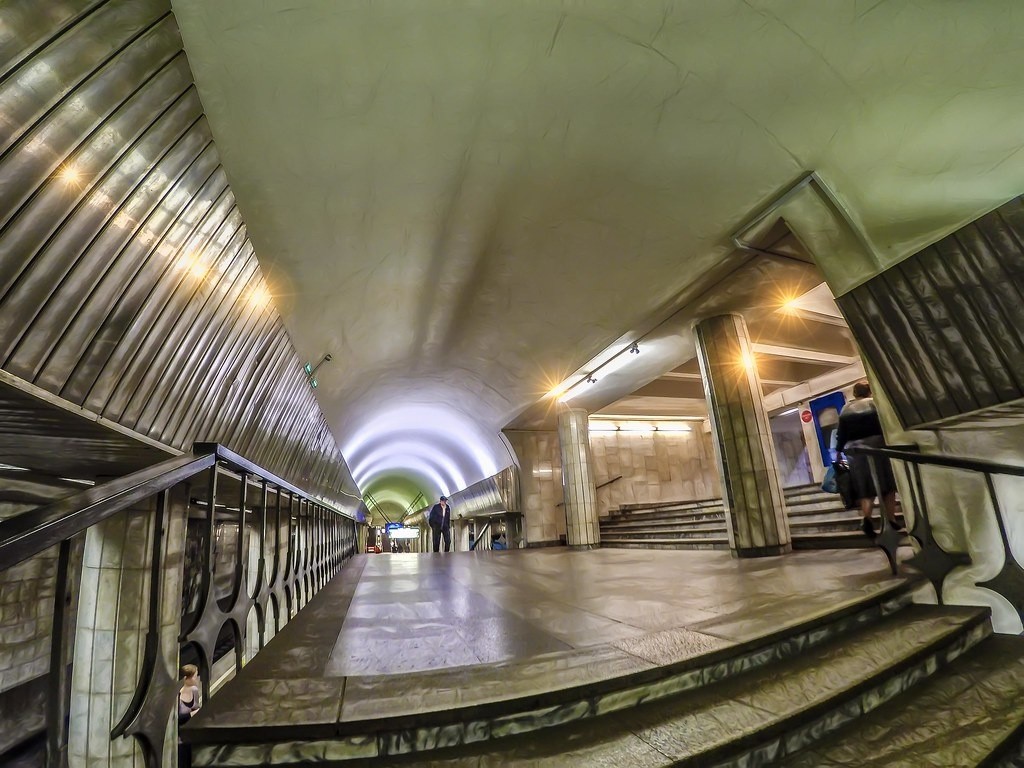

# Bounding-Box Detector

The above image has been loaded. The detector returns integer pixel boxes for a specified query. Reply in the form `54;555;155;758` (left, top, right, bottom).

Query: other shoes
888;519;902;531
861;516;877;535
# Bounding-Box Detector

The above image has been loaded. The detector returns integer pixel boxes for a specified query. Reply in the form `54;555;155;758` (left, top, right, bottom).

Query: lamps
630;343;640;354
587;375;596;384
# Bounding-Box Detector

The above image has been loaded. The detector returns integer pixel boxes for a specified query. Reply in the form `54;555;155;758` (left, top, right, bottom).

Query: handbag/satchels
821;456;840;494
832;461;862;511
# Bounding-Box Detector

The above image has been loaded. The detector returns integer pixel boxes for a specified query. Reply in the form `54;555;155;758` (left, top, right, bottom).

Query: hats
853;382;872;398
440;496;449;500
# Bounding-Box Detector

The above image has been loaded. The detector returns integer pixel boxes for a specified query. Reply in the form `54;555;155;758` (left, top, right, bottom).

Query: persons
835;380;902;536
177;664;200;768
374;542;410;554
429;495;451;552
